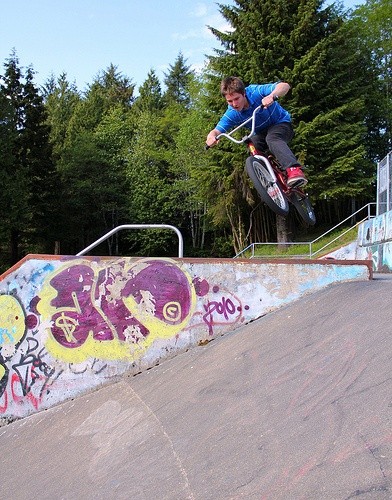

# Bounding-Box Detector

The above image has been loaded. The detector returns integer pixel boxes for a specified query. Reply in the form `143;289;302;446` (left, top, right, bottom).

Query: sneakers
286;167;308;187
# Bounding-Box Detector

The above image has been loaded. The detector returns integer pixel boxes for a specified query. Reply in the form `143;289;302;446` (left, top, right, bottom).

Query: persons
206;76;308;187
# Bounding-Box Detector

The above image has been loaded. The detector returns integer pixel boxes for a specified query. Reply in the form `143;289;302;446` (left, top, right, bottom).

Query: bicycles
204;94;316;225
